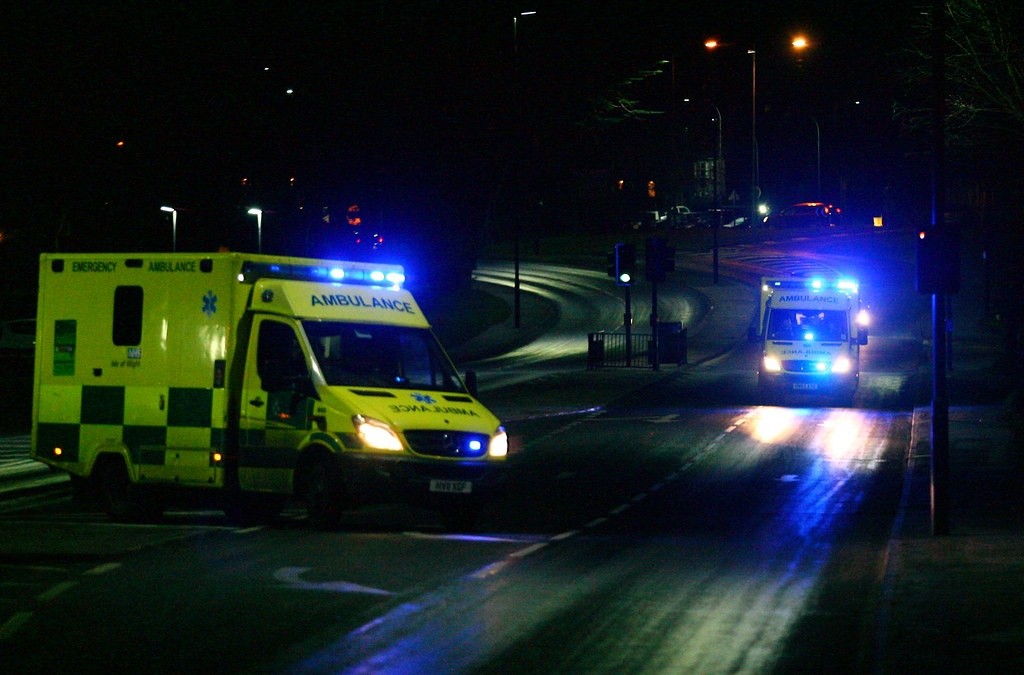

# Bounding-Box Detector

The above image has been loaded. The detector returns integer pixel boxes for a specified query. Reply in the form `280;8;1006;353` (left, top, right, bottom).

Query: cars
763;202;842;233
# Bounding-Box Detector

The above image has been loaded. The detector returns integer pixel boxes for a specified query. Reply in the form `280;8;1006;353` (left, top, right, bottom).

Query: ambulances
30;252;510;533
748;275;869;407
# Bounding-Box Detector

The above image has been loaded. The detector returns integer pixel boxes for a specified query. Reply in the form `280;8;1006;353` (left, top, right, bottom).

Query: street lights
704;34;809;250
160;207;177;252
514;11;538;57
246;208;262;250
684;97;718;283
